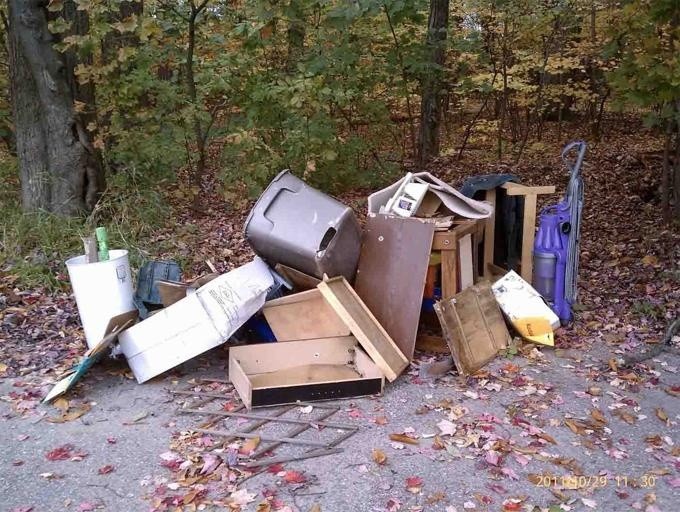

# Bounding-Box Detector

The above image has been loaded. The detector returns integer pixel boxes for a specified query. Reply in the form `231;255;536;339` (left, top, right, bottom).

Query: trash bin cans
65;248;134;350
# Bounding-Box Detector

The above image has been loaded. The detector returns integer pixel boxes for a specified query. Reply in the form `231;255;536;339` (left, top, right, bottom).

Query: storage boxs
242;168;361;285
